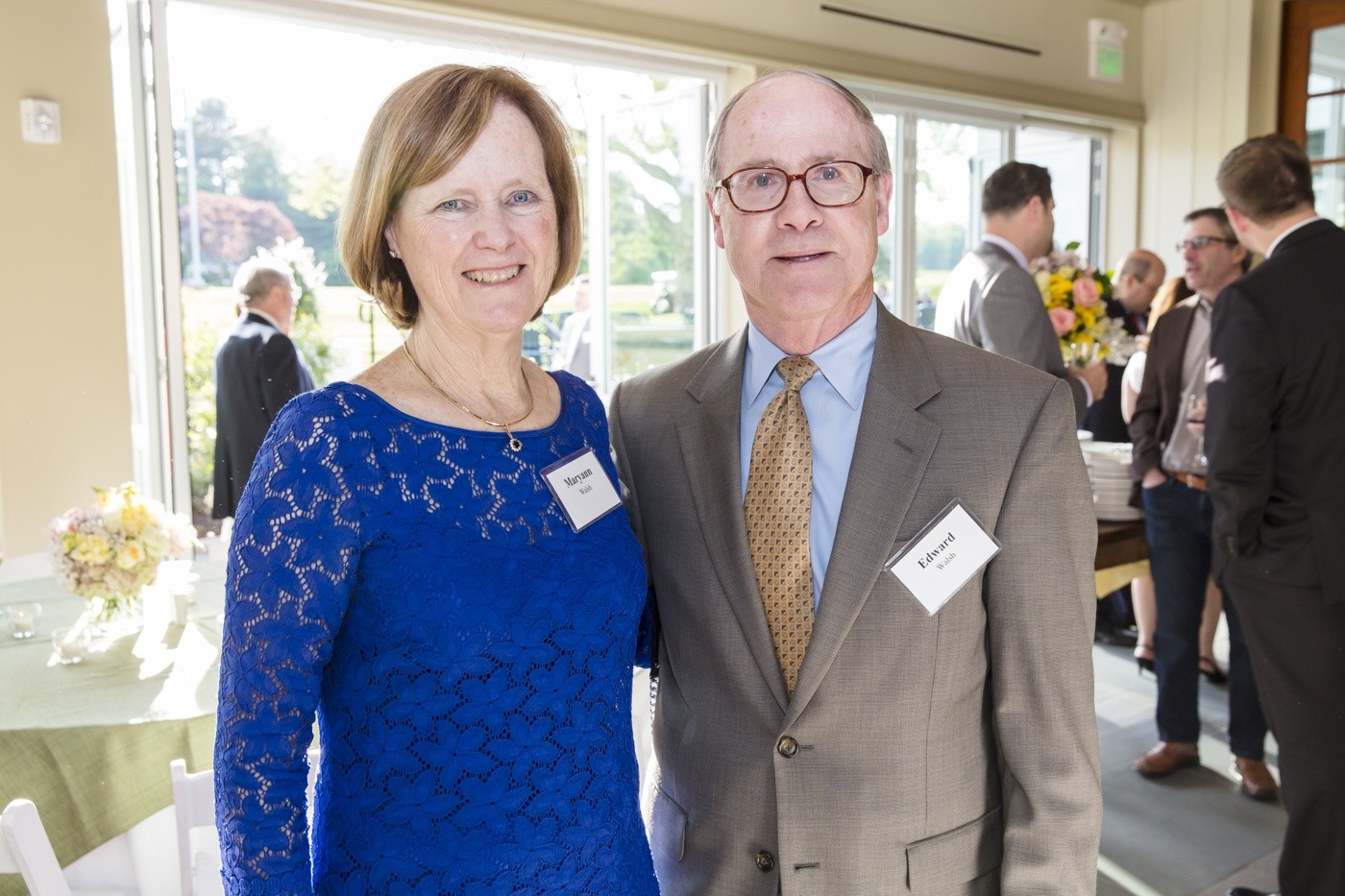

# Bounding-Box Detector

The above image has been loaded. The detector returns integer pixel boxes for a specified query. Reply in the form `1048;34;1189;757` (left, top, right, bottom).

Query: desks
1094;520;1149;571
1;553;216;896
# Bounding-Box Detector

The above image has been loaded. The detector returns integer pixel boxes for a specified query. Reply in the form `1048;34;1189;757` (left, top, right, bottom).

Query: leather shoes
1132;741;1199;776
1230;753;1276;800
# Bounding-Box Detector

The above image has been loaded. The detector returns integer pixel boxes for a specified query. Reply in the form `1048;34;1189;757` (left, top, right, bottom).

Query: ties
744;355;820;703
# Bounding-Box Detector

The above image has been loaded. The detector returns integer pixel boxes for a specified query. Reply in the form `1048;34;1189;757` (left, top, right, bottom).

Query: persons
935;161;1106;423
609;69;1104;896
1202;133;1345;896
210;257;316;527
1071;204;1278;803
212;64;661;896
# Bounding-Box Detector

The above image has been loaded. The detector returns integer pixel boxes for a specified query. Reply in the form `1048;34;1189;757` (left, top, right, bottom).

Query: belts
1167;471;1207;491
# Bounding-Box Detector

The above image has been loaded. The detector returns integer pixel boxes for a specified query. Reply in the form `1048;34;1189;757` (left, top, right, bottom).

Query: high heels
1199;657;1228;685
1134;644;1156;675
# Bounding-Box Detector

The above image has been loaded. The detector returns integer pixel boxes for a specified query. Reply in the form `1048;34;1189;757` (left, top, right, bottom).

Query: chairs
1;516;322;896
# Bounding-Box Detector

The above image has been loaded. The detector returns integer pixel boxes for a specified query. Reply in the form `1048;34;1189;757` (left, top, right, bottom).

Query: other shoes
1230;887;1270;896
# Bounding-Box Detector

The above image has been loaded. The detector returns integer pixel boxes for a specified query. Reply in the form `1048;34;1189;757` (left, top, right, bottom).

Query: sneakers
1095;620;1138;647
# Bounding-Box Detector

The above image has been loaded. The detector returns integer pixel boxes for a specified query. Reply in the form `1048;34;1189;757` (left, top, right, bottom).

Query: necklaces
403;341;534;452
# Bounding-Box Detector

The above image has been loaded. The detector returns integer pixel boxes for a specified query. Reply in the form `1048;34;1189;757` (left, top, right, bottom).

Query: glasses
1175;236;1231;251
716;160;880;213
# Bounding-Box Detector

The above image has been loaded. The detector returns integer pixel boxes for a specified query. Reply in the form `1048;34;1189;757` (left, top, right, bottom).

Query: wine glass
1187;394;1208;467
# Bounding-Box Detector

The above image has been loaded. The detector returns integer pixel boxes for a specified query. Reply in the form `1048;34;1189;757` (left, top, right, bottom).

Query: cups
1077;429;1093;443
1072;343;1100;369
53;626;90;664
4;603;42;638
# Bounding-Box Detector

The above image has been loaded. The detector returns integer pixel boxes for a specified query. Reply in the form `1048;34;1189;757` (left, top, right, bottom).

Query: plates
1077;443;1146;520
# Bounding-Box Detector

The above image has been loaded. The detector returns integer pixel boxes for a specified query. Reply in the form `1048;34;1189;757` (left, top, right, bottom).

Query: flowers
52;482;205;619
1028;241;1138;369
